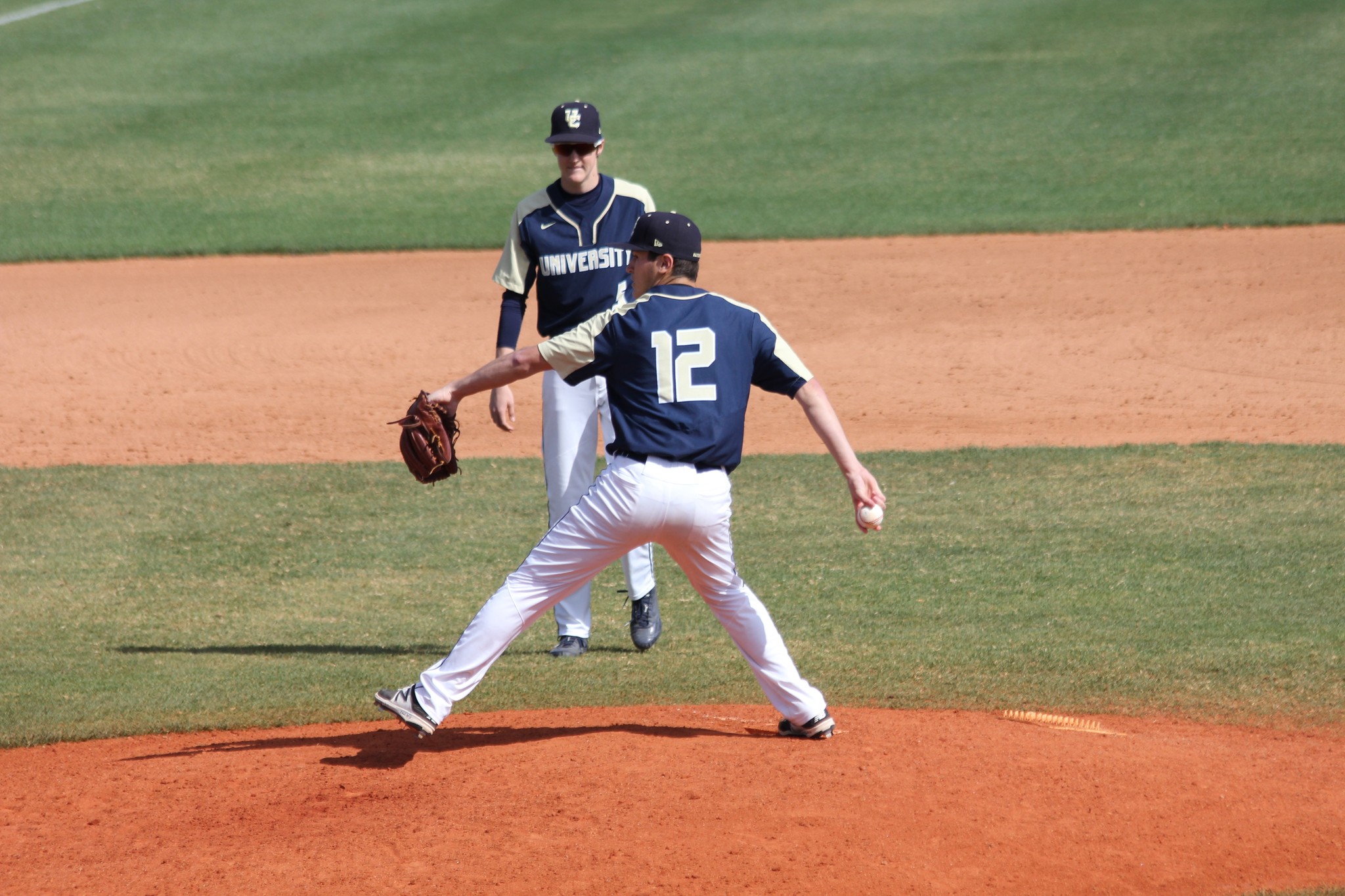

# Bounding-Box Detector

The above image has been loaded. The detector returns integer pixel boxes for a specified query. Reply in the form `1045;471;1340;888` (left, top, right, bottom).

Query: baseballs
855;504;884;530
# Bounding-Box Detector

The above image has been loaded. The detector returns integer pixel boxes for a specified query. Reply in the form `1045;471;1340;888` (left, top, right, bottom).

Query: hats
604;212;701;261
545;102;602;144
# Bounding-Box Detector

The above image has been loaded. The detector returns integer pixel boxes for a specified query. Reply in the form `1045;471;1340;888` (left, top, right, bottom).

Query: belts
615;450;721;471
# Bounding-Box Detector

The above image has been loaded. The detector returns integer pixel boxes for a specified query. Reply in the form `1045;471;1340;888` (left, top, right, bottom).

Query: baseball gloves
386;390;462;487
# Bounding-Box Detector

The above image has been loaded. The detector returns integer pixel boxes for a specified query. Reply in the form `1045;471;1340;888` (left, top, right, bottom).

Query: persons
371;210;886;738
490;103;661;655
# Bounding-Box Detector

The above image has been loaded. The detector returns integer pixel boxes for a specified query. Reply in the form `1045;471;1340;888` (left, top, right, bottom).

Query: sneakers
551;635;589;657
630;586;661;651
778;710;835;739
373;684;437;739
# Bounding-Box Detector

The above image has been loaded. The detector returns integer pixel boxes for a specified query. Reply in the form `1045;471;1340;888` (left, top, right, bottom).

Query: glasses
555;144;598;157
